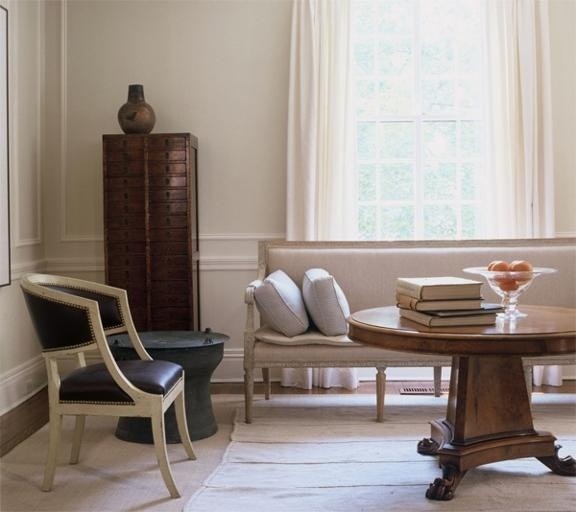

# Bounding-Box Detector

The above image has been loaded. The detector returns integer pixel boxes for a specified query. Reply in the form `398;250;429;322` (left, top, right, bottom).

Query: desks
106;331;231;444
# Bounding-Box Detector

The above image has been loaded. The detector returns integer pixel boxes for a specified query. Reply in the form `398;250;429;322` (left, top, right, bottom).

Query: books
396;277;507;328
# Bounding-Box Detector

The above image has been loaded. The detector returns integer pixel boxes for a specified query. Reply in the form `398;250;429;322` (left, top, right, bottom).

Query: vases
118;85;154;131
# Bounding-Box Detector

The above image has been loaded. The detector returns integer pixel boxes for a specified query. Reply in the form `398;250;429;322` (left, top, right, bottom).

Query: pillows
254;267;351;338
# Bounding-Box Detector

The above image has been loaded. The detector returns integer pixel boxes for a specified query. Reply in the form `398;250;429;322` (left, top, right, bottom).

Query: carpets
20;273;196;497
183;396;575;511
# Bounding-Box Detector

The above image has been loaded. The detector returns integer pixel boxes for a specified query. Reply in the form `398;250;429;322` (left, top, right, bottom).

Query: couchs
244;238;576;423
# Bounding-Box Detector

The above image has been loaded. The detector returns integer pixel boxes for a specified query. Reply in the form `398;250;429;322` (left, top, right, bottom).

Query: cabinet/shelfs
102;133;200;330
